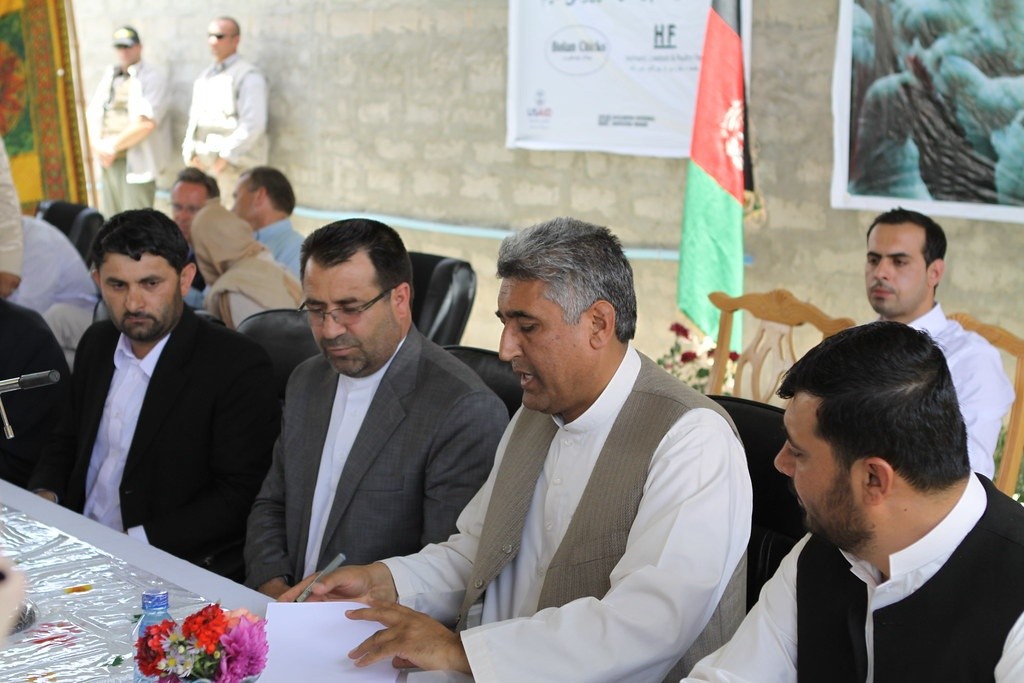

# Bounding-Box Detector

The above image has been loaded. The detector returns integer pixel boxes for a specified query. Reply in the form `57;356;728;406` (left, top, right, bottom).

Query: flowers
135;604;268;683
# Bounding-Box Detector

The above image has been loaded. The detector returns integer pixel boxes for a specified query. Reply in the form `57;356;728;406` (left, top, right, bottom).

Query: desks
0;480;473;683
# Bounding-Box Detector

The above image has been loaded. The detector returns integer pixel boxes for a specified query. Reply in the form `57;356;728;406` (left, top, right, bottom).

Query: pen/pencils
294;552;345;602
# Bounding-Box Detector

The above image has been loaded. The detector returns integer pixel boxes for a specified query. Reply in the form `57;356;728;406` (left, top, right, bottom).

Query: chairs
37;203;109;276
947;313;1024;498
707;393;810;611
452;347;525;419
707;292;857;405
236;308;323;417
400;248;479;349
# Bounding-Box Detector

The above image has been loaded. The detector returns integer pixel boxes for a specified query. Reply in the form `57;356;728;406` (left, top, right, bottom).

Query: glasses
207;31;237;40
297;288;396;327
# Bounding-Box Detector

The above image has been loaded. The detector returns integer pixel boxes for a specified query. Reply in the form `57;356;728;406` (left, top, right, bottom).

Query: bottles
132;590;176;683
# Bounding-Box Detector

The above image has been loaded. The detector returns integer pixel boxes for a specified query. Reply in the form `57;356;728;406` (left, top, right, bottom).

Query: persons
0;298;70;493
28;208;280;582
278;217;753;683
680;320;1024;683
170;167;221;308
181;17;271;210
244;219;510;601
867;207;1015;480
0;137;24;298
231;166;305;284
7;215;108;372
87;25;173;221
191;203;306;331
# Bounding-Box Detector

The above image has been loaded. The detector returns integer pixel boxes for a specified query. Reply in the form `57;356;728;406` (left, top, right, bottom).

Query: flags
676;0;755;357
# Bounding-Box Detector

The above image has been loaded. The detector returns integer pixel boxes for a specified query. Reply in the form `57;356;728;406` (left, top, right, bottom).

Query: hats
112;28;137;48
